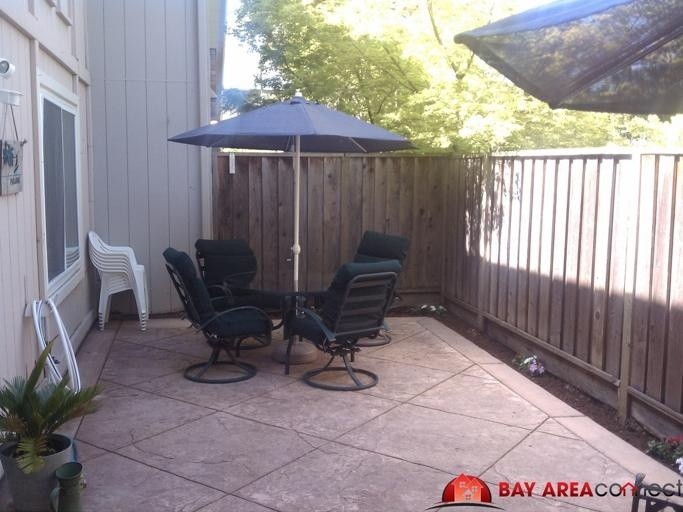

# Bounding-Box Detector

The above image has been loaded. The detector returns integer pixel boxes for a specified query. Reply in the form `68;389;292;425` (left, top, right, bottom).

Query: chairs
162;231;411;390
87;231;150;330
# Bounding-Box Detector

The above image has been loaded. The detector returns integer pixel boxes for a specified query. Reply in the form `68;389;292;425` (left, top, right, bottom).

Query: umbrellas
165;96;420;345
456;2;682;120
